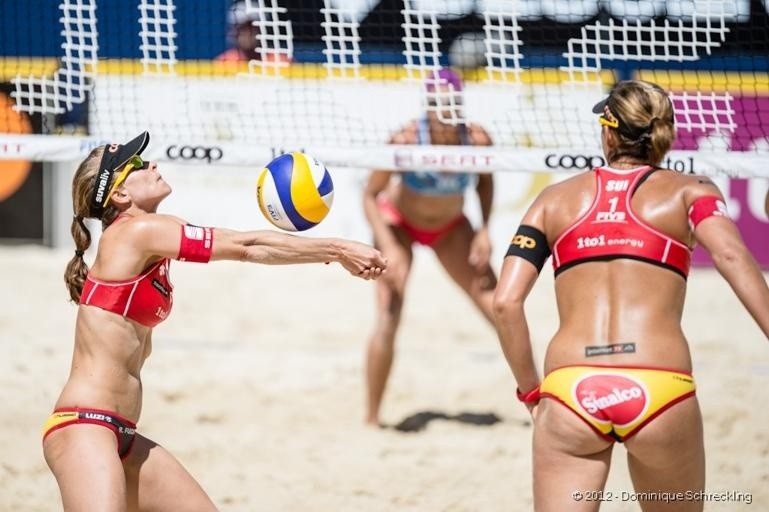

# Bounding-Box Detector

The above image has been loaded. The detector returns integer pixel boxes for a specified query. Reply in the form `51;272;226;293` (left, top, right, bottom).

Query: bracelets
512;385;541;403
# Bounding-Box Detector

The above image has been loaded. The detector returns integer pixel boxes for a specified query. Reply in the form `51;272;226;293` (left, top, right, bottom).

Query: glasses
103;155;143;208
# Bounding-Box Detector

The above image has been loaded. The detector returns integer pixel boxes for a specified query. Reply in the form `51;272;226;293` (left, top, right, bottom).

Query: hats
425;69;461;93
592;97;629;131
88;130;150;220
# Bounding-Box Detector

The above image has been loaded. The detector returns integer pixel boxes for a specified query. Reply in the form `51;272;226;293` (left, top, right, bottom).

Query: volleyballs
257;152;334;231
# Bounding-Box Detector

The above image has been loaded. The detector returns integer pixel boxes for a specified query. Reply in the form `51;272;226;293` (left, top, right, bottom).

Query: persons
358;65;501;433
210;1;297;64
494;78;768;512
38;127;394;509
